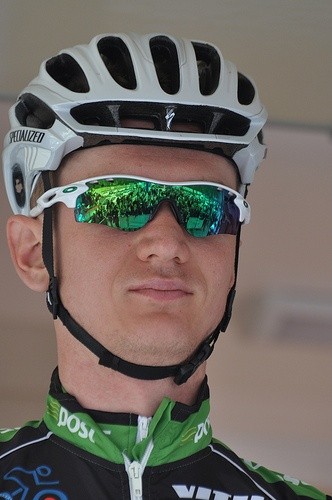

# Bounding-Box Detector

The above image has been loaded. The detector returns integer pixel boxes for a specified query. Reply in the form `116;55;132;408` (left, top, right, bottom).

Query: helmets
3;33;269;217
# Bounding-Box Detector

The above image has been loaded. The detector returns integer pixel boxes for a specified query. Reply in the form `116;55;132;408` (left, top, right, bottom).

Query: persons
0;32;332;500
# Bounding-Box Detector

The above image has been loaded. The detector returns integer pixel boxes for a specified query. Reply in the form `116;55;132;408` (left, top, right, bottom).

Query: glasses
29;173;252;238
